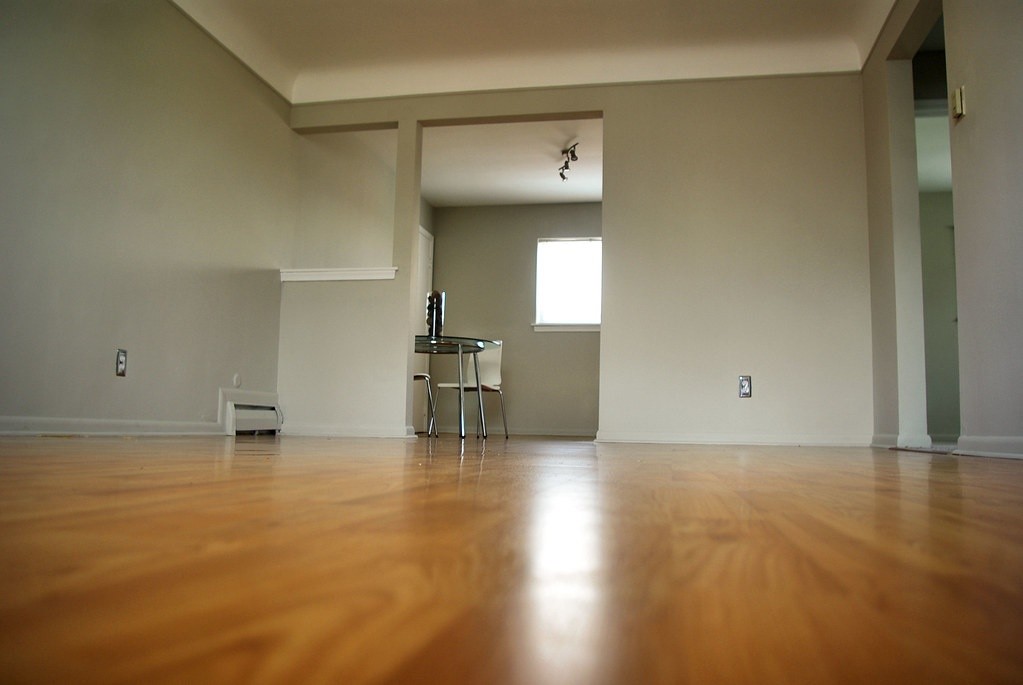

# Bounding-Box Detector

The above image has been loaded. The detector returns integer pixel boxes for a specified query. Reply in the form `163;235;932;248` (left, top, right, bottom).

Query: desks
414;335;502;439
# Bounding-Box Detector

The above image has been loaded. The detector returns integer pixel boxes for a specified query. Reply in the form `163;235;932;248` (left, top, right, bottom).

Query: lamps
570;146;578;161
564;152;571;172
559;168;568;182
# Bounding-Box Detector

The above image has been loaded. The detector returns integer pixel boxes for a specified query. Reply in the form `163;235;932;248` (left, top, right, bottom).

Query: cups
425;290;446;336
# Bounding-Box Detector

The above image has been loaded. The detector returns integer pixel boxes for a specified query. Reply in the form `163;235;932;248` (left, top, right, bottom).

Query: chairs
428;340;509;439
414;373;440;439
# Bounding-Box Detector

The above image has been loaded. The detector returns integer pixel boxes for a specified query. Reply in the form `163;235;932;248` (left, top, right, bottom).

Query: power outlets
739;375;753;398
116;349;128;378
